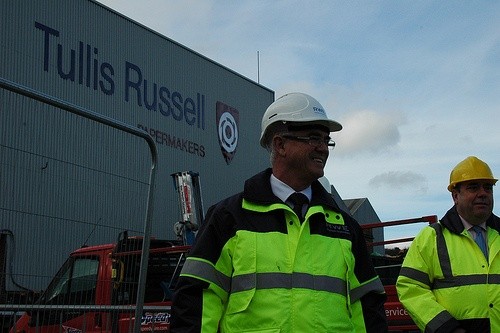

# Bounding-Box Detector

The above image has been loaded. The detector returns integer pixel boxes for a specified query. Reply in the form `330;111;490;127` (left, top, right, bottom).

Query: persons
170;92;389;333
396;156;500;333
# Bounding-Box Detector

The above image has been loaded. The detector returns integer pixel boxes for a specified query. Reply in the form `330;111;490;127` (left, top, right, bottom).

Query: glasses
281;134;335;150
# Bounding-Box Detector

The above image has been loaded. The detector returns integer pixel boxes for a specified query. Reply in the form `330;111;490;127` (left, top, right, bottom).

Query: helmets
259;92;343;148
447;156;498;192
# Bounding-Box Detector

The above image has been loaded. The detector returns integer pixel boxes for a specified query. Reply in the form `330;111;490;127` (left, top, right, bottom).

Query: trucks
9;215;439;333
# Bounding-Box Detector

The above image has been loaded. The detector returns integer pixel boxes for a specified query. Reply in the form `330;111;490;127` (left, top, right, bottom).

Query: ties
286;192;308;224
472;226;489;265
